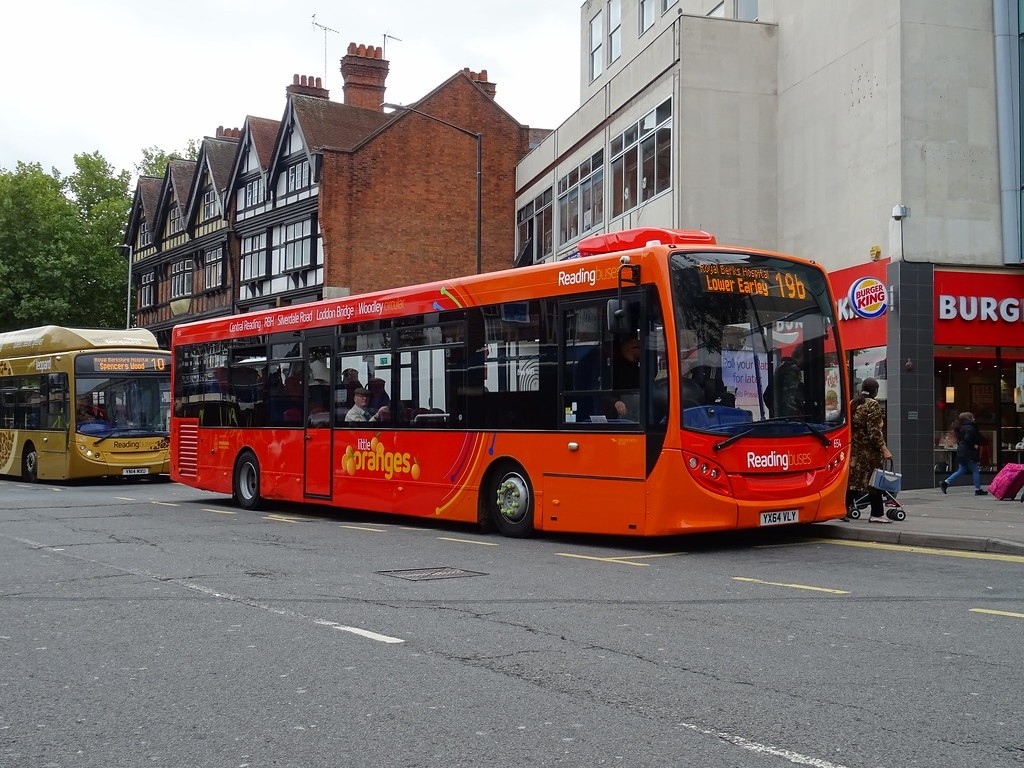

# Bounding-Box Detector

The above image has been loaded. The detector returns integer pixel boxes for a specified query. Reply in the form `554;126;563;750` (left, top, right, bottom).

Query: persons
272;352;390;425
840;378;893;524
939;412;988;496
595;336;654;419
763;342;805;421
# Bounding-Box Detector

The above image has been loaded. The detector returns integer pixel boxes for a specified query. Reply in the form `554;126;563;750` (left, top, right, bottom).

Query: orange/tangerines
342;446;356;477
411;464;420;480
371;437;384;455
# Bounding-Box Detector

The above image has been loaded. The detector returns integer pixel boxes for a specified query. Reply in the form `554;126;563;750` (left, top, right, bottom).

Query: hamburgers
826;391;839;410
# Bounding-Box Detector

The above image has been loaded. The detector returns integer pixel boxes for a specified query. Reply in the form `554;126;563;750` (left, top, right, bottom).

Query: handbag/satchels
868;458;902;492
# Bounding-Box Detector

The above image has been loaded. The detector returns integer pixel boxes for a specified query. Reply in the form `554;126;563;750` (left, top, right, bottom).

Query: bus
0;325;173;481
171;228;846;541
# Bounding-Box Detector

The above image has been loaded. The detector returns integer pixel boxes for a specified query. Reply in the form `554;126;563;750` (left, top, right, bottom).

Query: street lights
112;242;133;329
378;102;482;275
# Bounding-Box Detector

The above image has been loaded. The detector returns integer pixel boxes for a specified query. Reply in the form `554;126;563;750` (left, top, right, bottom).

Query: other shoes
939;481;948;494
841;516;850;522
975;489;988;495
869;515;893;523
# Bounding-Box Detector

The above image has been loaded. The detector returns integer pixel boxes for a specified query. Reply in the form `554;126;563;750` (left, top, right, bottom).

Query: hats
354;388;371;396
959;412;975;421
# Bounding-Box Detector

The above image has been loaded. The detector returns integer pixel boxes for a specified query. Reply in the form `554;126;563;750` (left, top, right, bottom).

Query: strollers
848;458;907;523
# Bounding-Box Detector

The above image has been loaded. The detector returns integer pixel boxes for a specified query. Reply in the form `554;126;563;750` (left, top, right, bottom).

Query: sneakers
886;500;903;507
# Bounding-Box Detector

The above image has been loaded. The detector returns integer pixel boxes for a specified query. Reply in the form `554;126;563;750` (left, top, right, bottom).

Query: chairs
214;365;445;426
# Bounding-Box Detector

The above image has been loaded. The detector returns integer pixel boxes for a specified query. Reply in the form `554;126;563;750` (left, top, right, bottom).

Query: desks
1002;449;1024;464
935;449;959;471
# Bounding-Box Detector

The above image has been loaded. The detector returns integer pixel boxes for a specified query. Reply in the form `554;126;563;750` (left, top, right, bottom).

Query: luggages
988;463;1024;501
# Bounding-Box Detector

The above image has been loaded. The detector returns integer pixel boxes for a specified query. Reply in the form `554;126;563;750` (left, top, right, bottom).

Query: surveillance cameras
892;206;905;220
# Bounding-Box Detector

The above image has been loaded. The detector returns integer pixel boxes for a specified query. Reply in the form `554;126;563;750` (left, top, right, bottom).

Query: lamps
945;364;955;403
